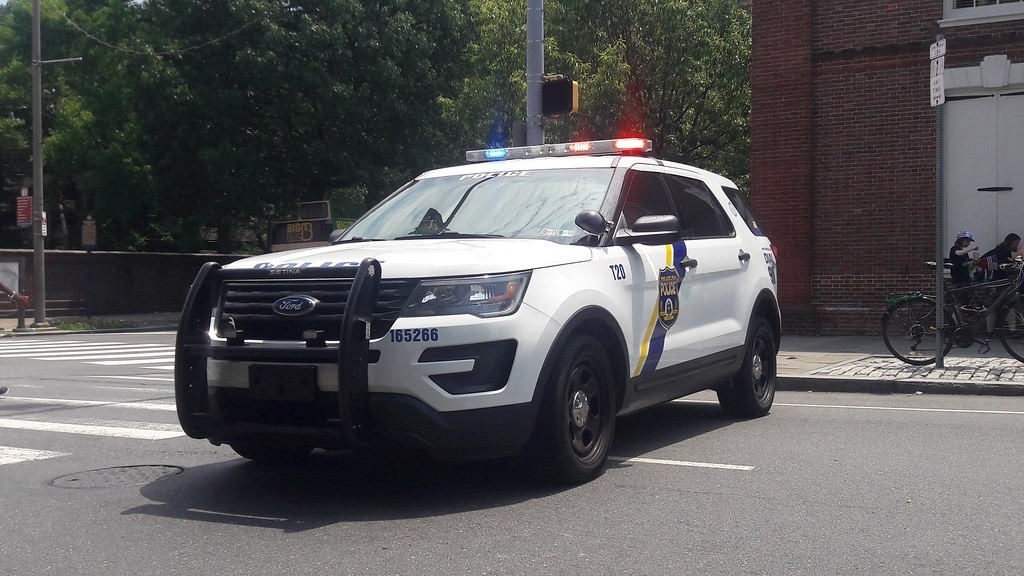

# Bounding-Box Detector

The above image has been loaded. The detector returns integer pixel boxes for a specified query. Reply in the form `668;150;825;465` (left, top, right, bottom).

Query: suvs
174;138;782;484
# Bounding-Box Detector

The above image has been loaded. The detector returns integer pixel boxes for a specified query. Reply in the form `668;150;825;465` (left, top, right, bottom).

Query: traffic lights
542;78;578;118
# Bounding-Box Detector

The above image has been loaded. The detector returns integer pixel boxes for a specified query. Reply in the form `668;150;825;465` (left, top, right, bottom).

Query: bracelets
8;291;16;298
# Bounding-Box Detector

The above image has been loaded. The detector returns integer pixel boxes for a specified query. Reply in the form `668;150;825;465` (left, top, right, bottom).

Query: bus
268;201;374;253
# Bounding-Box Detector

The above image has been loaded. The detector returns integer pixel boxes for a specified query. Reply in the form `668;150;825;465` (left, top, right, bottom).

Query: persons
972;233;1024;338
950;231;979;323
0;283;25;396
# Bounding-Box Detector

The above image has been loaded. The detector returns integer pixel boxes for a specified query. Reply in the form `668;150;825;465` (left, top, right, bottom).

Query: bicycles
882;253;1024;365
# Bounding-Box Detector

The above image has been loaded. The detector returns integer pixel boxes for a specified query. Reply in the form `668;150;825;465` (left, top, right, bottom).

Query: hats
956;230;975;242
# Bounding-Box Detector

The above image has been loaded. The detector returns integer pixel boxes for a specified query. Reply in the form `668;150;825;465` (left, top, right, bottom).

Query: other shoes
985;331;1002;339
0;387;9;396
1006;329;1022;338
